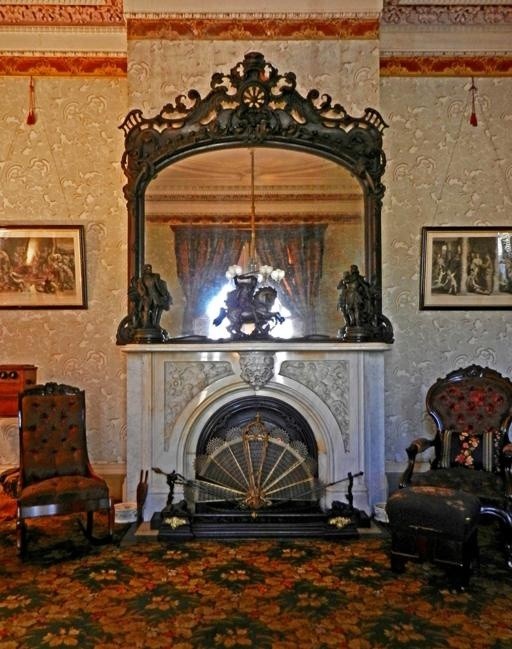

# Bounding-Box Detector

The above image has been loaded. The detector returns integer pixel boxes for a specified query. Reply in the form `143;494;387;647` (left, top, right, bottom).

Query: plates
1;363;39;418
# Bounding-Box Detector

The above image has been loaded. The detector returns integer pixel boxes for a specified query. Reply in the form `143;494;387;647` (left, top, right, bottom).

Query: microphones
384;484;478;597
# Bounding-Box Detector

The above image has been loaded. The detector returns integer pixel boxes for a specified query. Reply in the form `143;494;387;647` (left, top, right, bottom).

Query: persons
340;263;363;325
430;237;511;299
137;261;163;327
231;274;261;325
335;270;351;328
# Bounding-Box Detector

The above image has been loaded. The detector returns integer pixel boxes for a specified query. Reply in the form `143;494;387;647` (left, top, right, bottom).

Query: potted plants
0;224;89;310
418;225;512;312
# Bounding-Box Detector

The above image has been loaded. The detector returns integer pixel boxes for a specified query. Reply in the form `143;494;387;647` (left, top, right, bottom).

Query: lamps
398;364;512;564
16;380;114;569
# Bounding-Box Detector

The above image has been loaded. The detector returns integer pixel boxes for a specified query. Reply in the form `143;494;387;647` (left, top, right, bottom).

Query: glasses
222;149;287;287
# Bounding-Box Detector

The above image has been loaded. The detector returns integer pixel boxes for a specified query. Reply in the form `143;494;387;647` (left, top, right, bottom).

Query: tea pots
116;47;396;345
